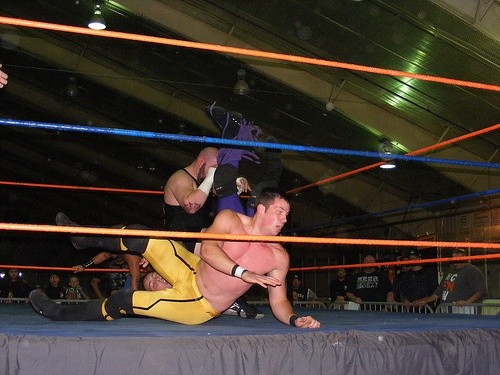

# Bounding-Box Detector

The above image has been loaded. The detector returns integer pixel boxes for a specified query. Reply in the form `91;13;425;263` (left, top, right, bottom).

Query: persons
136;99;265;320
0;252;155;304
329;269;349;303
29;188;322;329
382;249;486;315
292;274;317;309
345;253;396;312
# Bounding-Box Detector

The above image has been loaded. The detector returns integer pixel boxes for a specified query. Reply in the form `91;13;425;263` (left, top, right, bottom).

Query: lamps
326;79;346;111
380;145;396;169
86;0;106;30
234;67;249;95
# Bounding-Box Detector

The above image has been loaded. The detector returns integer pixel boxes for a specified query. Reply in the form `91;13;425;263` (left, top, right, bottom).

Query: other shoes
29;288;61;320
57;213;86;249
222;301;264;319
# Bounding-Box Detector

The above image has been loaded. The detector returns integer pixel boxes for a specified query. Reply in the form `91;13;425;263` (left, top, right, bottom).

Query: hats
452;248;466;254
338;268;345;271
292;274;300;281
408;249;422;259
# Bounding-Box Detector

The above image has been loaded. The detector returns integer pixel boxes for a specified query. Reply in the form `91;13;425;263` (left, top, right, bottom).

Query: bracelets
232;264;247;278
290;314;298;327
